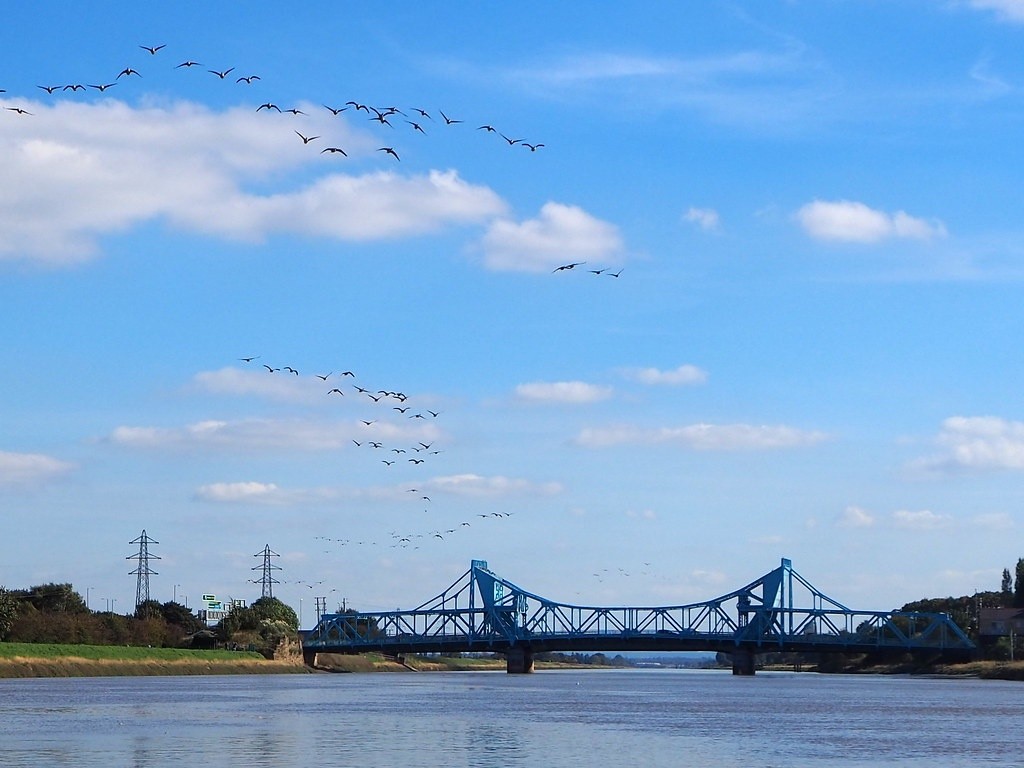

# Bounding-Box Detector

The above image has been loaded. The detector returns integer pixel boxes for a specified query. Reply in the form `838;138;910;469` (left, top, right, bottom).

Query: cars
622;628;640;634
395;633;413;640
518;626;536;637
658;630;676;634
683;627;696;634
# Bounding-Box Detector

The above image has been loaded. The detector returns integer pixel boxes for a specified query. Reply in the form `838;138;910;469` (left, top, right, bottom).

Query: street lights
87;586;95;609
101;598;109;612
111;599;118;619
300;598;304;630
179;595;188;609
174;584;181;602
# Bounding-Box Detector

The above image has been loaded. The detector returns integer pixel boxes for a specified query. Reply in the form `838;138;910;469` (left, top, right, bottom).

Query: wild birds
552;262;625;278
238;355;513;588
0;44;545;161
576;563;651;594
